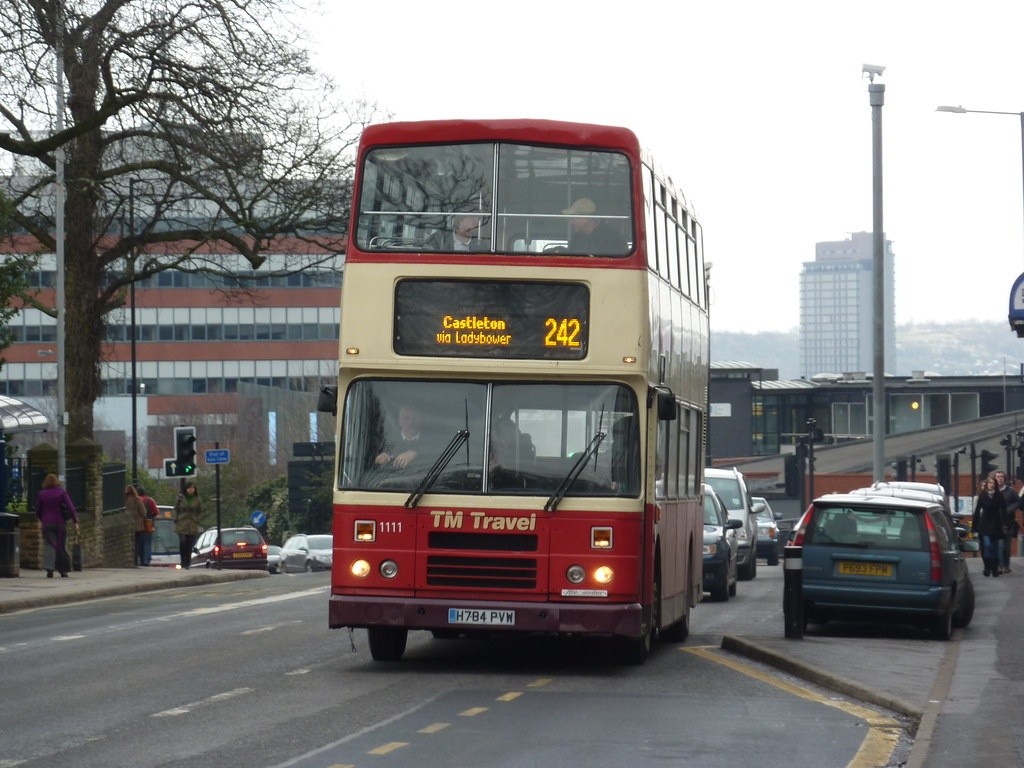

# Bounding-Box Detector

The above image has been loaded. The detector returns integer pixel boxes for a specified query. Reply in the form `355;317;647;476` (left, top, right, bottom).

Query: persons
425;213;487;252
374;404;426;469
124;482;161;566
172;484;202;570
36;475;80;577
971;470;1024;577
561;198;628;255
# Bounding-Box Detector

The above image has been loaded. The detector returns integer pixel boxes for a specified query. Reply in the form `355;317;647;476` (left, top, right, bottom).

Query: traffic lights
164;426;199;478
979;450;1000;489
891;456;907;482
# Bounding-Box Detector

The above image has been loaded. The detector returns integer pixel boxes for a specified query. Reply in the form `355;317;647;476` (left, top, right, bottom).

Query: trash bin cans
0;512;21;577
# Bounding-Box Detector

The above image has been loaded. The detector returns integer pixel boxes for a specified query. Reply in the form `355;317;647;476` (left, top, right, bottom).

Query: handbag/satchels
1010;520;1019;538
61;491;70;522
998;510;1007;539
72;534;83;572
144;495;155;533
173;493;182;523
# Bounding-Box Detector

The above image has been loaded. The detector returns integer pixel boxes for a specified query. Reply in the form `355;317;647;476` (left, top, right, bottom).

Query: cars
750;496;782;563
267;544;284;573
653;480;744;602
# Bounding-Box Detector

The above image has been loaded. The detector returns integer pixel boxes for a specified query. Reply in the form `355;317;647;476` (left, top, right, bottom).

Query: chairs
823;518;857;544
901;516;920;547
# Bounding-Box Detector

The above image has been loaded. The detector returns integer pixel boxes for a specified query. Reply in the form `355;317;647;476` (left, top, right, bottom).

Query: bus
316;119;714;659
104;506;183;568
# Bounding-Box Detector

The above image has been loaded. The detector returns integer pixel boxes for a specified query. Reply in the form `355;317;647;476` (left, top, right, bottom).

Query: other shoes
983;569;990;576
1004;567;1011;573
999;569;1003;574
61;572;68;577
47;572;53;578
992;570;999;577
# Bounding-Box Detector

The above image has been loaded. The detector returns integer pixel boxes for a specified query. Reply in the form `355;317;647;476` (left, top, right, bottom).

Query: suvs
278;533;333;573
702;467;766;580
849;479;966;545
779;492;980;639
188;525;270;570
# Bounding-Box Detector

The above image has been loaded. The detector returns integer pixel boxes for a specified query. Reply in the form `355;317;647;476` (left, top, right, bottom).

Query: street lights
953;447;968;513
861;63;890;489
1001;432;1015;485
938;103;1023;240
910;454;927;483
807;417;823;503
